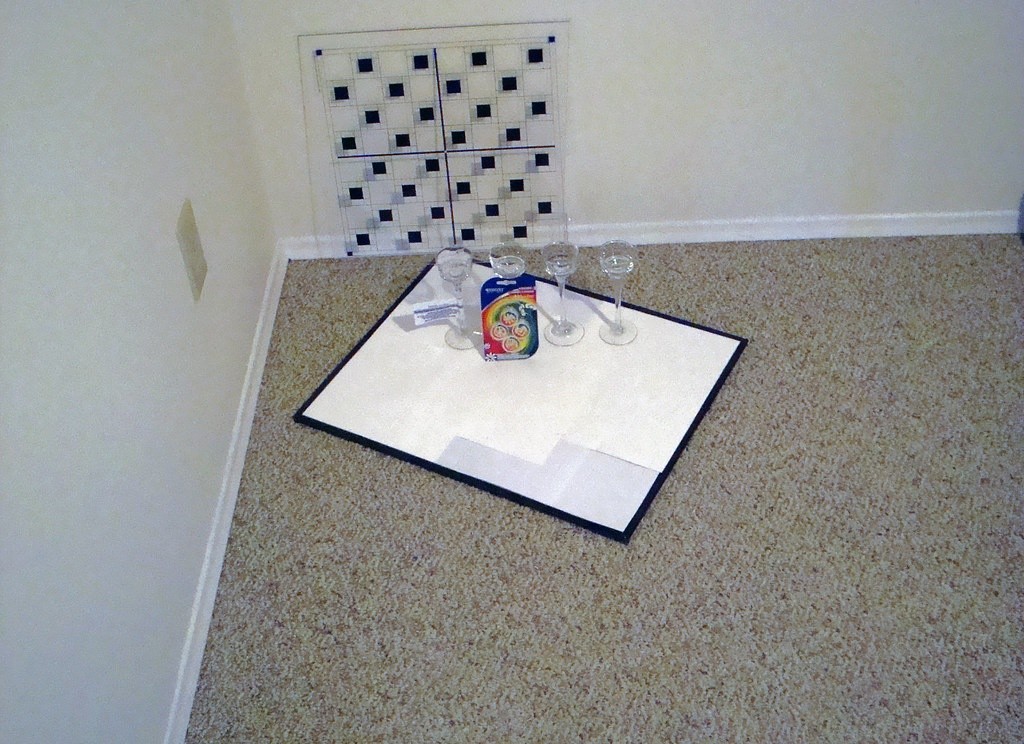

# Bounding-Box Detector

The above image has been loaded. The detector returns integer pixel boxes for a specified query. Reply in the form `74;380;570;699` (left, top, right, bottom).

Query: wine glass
436;247;483;349
540;242;584;347
600;240;639;345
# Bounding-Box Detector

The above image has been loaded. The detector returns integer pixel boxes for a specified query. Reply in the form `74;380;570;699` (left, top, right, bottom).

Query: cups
489;243;527;278
